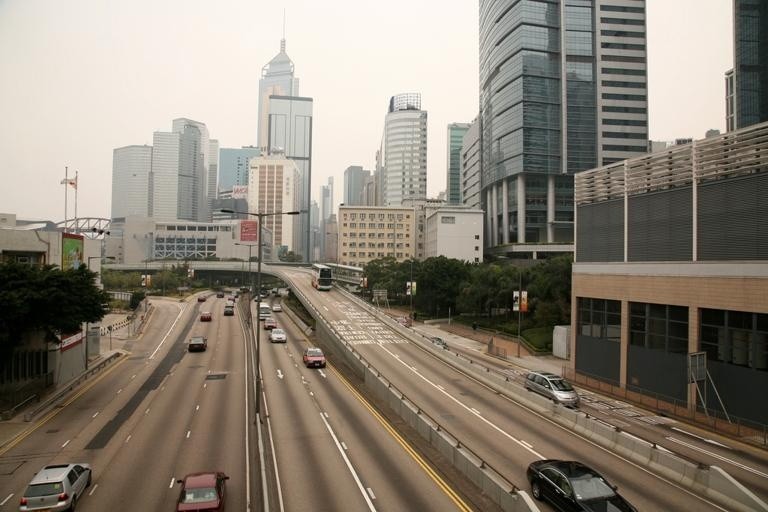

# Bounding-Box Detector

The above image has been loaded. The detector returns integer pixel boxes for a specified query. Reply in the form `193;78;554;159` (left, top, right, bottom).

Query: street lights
509;264;522;357
407;263;412;324
221;209;300;413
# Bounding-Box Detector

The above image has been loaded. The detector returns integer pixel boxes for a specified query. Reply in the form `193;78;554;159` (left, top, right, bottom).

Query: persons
414;311;417;320
472;322;477;331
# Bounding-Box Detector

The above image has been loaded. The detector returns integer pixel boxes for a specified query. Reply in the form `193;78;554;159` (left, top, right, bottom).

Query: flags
60;178;77;189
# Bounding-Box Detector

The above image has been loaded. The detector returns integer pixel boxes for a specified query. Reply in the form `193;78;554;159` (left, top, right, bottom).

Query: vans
525;372;580;406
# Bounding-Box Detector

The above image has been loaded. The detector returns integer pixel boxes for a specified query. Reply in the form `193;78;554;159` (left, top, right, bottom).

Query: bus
257;303;272;320
312;263;364;290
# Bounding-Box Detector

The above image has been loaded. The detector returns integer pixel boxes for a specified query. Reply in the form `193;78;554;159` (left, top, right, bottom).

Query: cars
527;459;636;511
176;471;228;511
198;285;286;343
188;336;207;351
304;348;325;368
19;464;92;512
